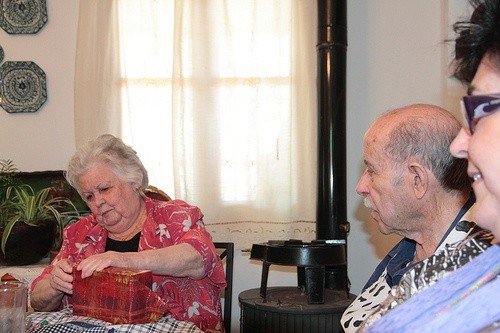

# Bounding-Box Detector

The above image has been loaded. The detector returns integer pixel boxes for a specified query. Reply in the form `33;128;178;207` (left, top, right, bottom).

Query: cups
0;282;27;333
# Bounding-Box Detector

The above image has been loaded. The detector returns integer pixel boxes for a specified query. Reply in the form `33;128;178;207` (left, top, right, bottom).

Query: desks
238;286;358;333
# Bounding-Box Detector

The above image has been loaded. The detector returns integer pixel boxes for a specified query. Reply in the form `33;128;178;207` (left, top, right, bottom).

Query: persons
339;103;498;333
30;134;228;333
357;0;500;332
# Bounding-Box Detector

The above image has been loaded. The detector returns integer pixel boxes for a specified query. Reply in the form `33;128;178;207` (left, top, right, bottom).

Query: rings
51;274;56;278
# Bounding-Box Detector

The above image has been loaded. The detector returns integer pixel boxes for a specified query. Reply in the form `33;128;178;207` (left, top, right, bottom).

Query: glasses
459;91;500;134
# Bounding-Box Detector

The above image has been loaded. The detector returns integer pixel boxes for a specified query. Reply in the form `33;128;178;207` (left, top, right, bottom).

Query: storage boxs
71;266;153;325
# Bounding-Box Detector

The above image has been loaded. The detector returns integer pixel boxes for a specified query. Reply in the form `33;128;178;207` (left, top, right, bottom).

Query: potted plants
0;184;80;265
